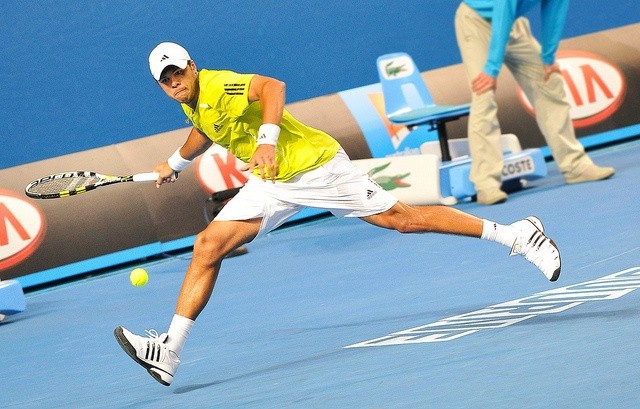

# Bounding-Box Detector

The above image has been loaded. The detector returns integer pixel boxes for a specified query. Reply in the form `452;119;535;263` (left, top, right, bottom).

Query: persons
113;42;560;386
455;0;614;206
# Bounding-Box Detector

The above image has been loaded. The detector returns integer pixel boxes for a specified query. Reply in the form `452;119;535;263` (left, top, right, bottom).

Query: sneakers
510;215;561;282
564;164;615;183
477;187;508;204
114;326;181;386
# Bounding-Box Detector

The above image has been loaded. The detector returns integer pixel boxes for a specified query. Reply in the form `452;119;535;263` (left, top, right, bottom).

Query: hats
148;42;191;81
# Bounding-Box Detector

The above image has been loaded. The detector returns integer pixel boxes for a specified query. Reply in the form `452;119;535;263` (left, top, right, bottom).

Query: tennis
130;268;149;288
